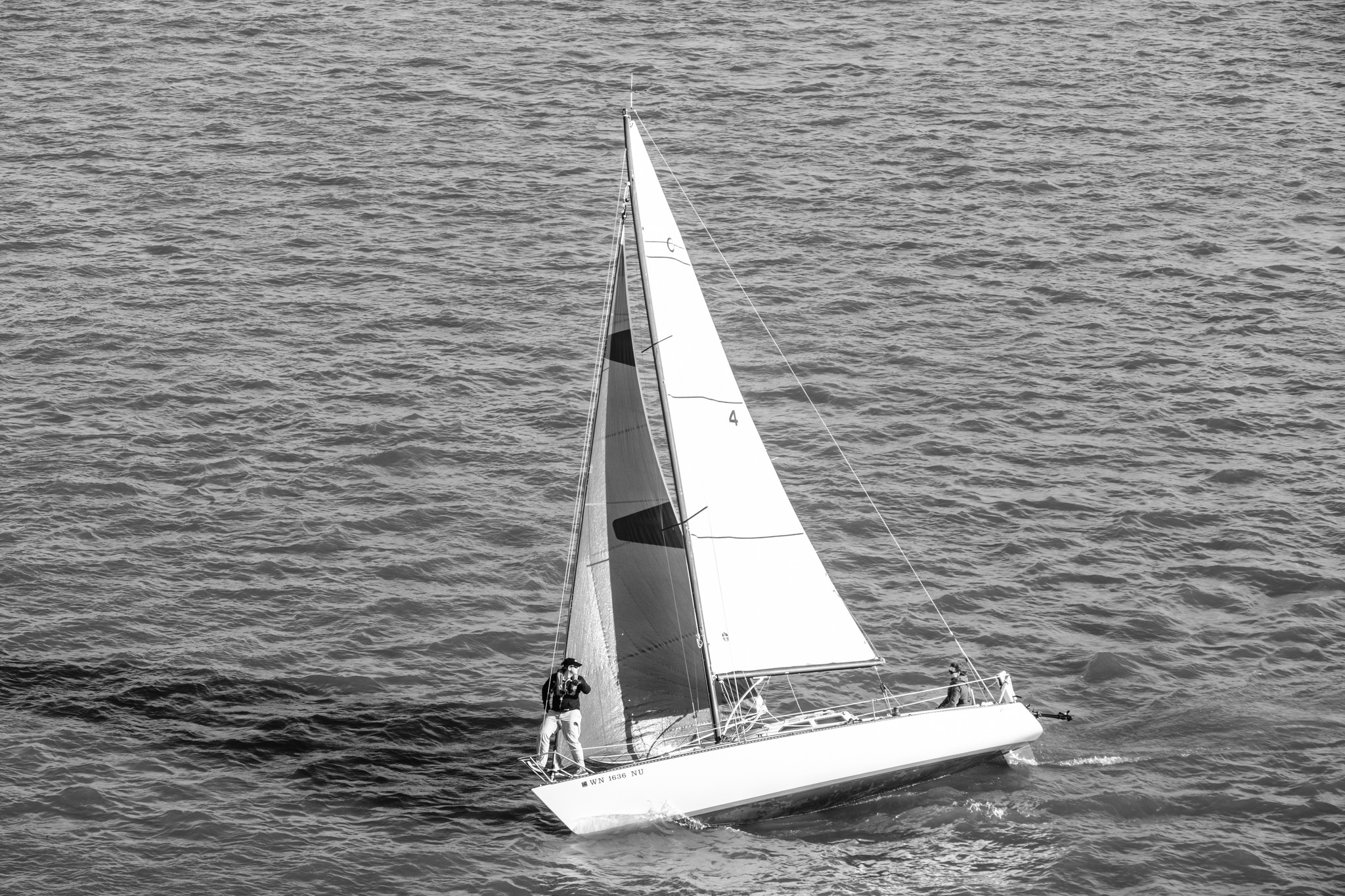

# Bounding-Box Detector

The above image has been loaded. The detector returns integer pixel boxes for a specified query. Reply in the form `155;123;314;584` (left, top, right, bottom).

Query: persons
526;657;591;775
935;662;972;710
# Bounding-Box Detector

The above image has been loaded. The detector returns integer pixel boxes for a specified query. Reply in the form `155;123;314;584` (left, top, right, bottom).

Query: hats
563;658;582;667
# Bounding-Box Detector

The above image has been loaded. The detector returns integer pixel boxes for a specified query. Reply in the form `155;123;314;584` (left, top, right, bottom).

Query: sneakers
527;761;544;773
573;767;589;777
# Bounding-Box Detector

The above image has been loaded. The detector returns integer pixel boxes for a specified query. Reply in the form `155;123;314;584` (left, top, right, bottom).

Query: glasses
948;669;957;674
568;665;578;668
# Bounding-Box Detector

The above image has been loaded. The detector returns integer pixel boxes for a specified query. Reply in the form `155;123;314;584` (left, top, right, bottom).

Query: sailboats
519;72;1078;842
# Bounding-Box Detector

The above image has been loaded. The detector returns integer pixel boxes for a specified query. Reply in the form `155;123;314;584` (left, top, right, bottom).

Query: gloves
572;679;582;686
544;705;551;713
